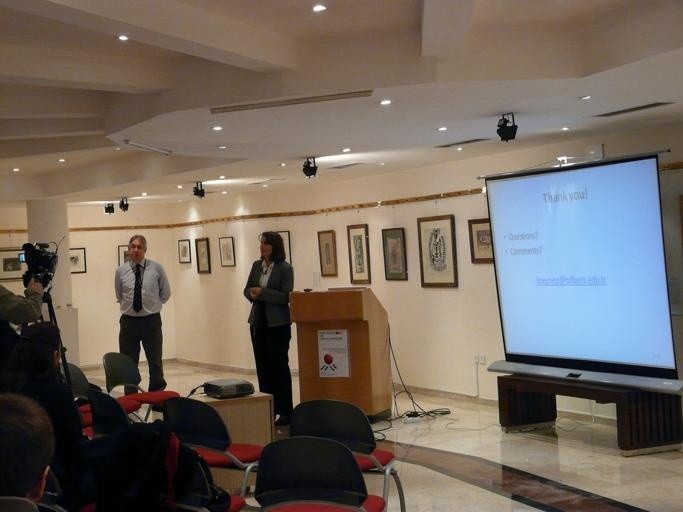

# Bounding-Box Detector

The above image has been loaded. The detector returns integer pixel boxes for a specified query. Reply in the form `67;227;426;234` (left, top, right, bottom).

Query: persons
244;231;294;427
0;278;85;512
115;235;171;397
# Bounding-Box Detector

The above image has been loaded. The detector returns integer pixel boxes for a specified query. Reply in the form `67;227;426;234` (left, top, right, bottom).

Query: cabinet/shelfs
495;372;682;459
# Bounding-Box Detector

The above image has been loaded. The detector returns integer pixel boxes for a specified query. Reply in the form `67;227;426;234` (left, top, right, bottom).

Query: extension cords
404;413;421;423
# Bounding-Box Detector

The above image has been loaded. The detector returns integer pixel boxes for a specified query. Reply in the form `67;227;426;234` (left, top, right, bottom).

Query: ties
133;264;143;314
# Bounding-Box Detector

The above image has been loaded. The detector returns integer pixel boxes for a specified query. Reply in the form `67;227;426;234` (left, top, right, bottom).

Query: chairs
253;435;386;511
1;351;145;511
103;351;180;423
169;446;246;511
290;399;408;511
163;395;264;498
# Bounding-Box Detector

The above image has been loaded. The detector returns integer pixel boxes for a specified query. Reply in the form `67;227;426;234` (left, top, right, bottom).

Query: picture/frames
69;247;86;274
277;230;292;267
117;245;129;267
315;212;495;290
0;249;27;282
176;235;238;282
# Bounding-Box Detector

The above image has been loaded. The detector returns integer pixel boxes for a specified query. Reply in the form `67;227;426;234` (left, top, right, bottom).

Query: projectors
205;379;255;398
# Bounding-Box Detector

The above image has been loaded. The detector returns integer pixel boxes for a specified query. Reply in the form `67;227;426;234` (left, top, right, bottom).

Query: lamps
495;108;517;144
103;202;115;215
192;181;205;199
117;196;129;213
301;155;317;179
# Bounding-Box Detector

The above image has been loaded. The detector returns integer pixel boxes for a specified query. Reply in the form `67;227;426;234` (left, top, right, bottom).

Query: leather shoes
274;414;293;427
